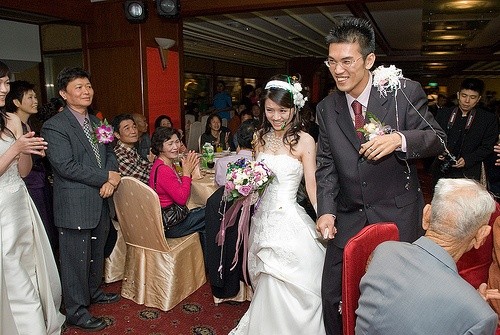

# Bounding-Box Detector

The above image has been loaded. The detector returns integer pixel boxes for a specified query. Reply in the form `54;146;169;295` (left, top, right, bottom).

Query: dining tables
179;161;219;210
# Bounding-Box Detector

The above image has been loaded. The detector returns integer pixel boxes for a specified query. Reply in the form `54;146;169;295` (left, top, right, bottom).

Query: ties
81;119;103;169
350;101;366;140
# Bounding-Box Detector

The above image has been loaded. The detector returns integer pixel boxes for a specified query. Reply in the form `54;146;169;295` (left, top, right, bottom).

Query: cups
175;161;183;176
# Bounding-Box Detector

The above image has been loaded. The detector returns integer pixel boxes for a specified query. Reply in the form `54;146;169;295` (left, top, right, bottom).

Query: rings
108;189;111;192
369;147;374;152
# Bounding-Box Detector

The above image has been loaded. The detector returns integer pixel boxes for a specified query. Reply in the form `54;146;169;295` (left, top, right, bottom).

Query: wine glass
207;159;215;177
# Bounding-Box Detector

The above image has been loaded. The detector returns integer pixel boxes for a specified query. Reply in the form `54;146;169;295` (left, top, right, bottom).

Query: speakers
124;0;147;23
156;0;180;19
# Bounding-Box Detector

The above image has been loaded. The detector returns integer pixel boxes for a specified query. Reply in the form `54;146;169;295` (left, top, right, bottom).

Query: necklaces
265;131;285;153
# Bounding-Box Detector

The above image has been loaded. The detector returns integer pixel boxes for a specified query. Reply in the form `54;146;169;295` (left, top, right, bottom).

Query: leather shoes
96;293;120;304
69;316;108;331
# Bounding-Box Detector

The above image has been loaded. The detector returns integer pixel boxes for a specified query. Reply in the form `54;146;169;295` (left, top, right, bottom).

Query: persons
208;79;337;147
6;80;65;281
200;113;237;152
40;67;122;331
149;126;209;274
132;114;159;163
112;113;153;186
214;119;262;188
0;60;66;335
149;115;187;156
355;177;500;335
231;16;449;335
422;77;500;202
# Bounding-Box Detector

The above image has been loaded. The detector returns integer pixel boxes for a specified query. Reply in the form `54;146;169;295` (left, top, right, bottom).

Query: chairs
100;174;205;308
448;200;500;326
182;113;234;150
337;220;404;335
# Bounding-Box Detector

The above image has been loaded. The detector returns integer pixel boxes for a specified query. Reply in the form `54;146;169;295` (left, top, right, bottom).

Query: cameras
439;152;458;177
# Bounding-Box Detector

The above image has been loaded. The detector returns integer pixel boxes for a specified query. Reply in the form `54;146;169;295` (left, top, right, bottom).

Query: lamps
157;0;179;17
154;36;177;72
123;0;150;24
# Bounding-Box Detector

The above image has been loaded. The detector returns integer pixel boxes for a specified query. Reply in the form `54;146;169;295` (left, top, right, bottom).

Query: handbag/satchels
161;202;191;231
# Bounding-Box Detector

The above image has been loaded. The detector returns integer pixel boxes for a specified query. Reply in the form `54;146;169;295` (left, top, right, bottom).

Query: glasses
323;56;363;70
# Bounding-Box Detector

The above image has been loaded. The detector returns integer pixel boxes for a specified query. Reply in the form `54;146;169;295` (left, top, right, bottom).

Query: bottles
211;141;222;153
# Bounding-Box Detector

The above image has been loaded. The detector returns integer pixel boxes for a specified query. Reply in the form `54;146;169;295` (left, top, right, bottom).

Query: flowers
290;82;307;110
222;158;271;200
355;111;393;143
89;120;118;144
